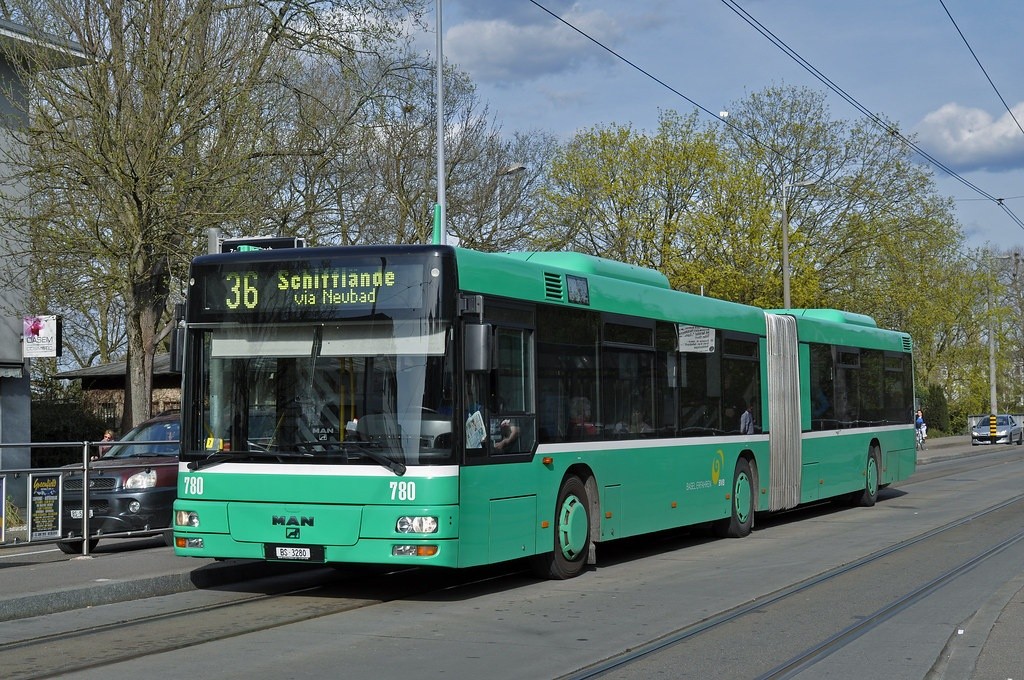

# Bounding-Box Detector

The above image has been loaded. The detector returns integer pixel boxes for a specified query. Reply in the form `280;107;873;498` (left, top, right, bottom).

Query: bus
170;241;920;582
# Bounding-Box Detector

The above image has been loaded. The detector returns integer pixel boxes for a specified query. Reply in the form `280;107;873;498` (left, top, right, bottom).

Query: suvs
53;410;220;555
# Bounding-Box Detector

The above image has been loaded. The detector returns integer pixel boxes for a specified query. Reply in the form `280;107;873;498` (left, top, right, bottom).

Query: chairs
264;378;905;456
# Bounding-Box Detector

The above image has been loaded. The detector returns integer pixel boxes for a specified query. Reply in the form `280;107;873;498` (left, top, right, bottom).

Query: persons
96;430;116;457
319;368;375;430
425;377;481;422
157;430;178;452
569;397;594;436
612;402;653;434
491;397;521;450
739;404;755;435
914;409;928;445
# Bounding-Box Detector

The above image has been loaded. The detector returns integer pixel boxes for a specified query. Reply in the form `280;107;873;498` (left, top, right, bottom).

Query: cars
970;415;1024;445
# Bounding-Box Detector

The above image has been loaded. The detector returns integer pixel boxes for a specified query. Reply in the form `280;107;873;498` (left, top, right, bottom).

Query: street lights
718;110;816;310
493;163;530;248
